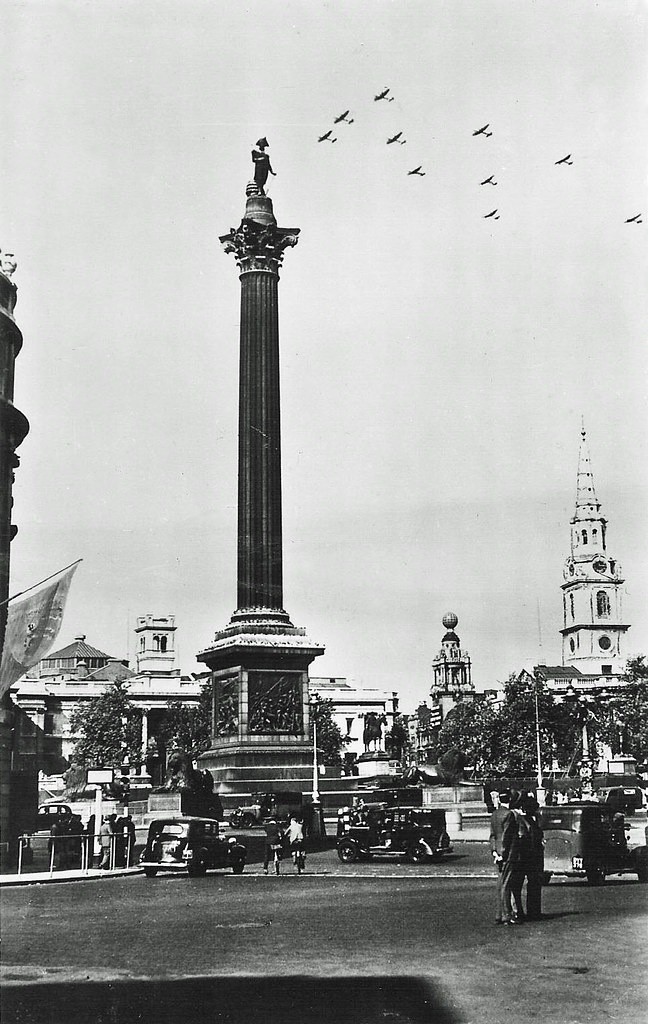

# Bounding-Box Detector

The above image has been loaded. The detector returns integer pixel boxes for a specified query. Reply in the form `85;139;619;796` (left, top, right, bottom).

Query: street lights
303;692;328;839
560;684;614;801
522;681;550;808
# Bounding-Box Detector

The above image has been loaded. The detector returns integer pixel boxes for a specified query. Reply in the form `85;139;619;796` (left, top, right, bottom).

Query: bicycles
269;836;284;875
285;834;307;875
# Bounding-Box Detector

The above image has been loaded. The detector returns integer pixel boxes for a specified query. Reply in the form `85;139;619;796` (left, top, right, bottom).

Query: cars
594;786;647;816
536;799;648;886
335;802;454;864
137;816;247;878
37;804;82;831
228;792;307;830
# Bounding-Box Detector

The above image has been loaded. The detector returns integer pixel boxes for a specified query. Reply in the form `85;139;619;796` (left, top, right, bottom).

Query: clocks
592;560;607;573
568;564;574;575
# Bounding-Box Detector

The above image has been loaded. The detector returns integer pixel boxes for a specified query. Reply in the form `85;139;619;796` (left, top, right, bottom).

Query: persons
490;787;598;927
336;797;369;841
262;811;306;874
252;137;278;196
99;813;136;869
366;712;376;728
49;813;95;868
